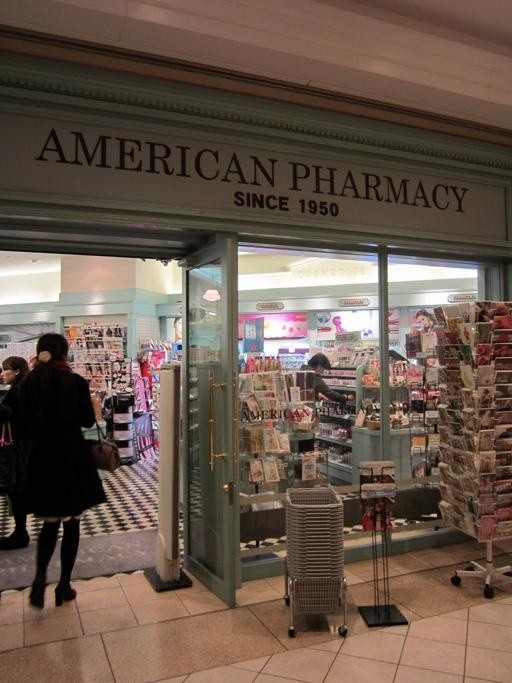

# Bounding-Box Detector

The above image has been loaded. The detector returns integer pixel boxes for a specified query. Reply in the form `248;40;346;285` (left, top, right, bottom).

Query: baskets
281;484;347;615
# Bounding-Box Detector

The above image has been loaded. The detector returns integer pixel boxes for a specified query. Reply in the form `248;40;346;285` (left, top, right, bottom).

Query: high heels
54;582;78;606
28;576;46;609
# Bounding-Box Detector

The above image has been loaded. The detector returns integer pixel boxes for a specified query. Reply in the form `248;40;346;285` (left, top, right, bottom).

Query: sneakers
0;529;30;550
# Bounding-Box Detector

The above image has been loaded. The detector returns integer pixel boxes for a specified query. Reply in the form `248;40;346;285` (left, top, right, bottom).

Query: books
434;299;511;541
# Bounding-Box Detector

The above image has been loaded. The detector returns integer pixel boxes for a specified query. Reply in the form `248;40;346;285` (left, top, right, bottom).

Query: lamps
203;263;222;302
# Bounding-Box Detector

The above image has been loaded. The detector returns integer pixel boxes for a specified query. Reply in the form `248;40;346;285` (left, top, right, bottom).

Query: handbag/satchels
84;416;122;473
0;421;20;481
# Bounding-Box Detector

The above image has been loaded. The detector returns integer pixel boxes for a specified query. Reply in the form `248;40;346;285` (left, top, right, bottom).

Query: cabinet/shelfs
317;348;425;485
106;396;134;466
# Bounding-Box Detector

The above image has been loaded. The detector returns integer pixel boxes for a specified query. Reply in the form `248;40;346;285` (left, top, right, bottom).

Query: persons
0;355;32;551
1;331;107;608
415;307;436;330
296;350;354;473
173;317;182;341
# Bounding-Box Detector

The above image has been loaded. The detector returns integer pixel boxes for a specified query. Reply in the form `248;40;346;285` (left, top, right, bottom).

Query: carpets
0;528;158;594
